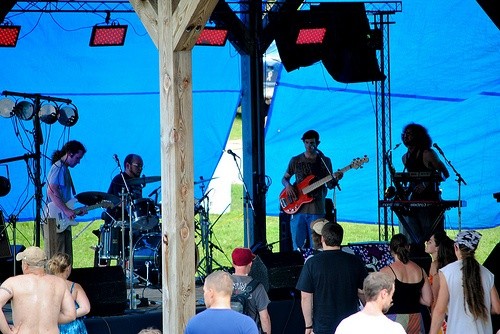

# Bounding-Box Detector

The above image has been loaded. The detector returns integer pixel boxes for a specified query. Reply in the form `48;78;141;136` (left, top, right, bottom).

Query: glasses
131;163;143;168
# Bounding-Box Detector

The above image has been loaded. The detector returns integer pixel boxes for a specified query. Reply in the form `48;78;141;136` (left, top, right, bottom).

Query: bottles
130;290;137;309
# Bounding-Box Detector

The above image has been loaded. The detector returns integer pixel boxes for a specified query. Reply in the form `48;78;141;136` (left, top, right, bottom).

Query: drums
127;198;159;230
98;221;130;259
133;232;198;289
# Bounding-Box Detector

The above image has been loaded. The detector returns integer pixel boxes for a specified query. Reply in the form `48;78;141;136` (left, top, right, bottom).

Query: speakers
264;251;307;300
6;245;26;276
71;266;127;315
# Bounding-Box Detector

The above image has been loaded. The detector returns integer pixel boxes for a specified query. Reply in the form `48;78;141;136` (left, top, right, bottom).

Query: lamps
362;28;383;50
294;14;328;44
0;19;21;48
193;17;229;47
90;11;128;47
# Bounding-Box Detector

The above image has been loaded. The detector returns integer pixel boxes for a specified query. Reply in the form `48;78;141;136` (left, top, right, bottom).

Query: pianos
379;199;467;247
390;170;450;200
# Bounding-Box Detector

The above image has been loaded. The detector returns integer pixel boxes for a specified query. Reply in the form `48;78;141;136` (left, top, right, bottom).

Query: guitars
43;199;112;233
280;155;369;214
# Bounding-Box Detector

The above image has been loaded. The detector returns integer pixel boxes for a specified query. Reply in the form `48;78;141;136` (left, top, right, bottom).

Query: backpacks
231;278;262;330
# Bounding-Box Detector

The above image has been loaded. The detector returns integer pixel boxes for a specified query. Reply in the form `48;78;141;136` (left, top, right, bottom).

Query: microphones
227;150;240;158
311;145;321;154
393;143;402;150
433;143;443;153
200;176;204;184
113;154;120;165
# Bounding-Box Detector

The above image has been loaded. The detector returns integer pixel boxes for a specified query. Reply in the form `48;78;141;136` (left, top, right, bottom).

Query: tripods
113;161;154;286
195;188;235;285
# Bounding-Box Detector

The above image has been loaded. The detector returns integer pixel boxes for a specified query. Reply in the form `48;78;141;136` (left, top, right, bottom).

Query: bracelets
306;326;313;329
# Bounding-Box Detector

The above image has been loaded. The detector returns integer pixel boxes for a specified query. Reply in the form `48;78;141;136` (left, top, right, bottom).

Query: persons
399;123;449;244
185;270;259;334
106;154;143;208
46;252;90;334
379;230;500;334
0;246;77;334
45;140;88;267
296;218;369;334
138;328;161;334
230;248;271;334
282;130;343;251
335;272;407;334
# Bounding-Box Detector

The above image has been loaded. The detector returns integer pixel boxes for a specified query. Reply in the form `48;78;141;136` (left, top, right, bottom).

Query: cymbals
194;176;219;185
122;176;161;186
74;191;122;208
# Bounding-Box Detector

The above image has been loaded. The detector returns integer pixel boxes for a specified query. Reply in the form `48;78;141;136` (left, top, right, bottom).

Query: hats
15;246;47;262
232;248;256;266
310;218;330;236
